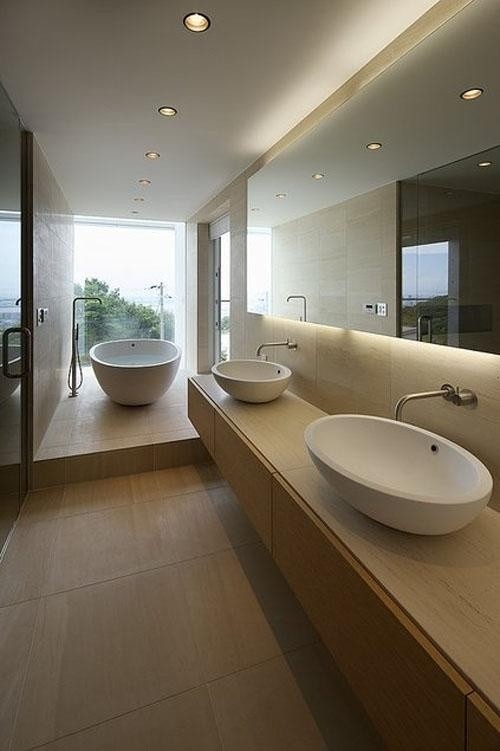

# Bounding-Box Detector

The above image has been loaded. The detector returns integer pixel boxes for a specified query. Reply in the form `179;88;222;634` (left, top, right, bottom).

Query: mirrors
246;1;499;355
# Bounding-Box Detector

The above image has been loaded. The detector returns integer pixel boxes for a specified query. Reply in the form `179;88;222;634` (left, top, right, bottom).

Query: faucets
286;296;307;322
254;338;290;356
70;297;103;397
393;384;456;422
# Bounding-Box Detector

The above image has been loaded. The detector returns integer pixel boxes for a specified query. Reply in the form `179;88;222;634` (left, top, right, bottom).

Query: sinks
303;414;495;536
211;359;293;403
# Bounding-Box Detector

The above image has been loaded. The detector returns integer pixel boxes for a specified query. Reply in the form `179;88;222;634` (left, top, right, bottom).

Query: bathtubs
88;338;181;406
